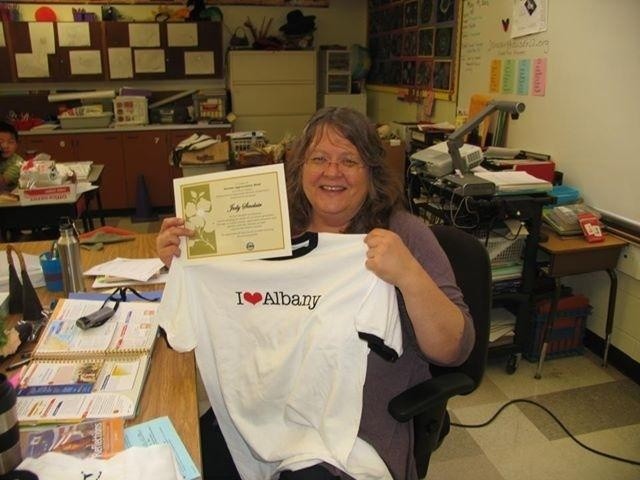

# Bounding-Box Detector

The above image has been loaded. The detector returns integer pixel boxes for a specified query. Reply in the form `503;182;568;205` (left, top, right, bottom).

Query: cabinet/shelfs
26;131;129;215
102;13;225;84
0;15;18;88
9;15;108;85
322;92;371;125
380;137;408;205
222;43;319;147
322;44;354;99
118;123;233;212
14;131;29;161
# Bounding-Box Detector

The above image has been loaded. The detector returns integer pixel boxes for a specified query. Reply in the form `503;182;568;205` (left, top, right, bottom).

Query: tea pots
229;26;249;47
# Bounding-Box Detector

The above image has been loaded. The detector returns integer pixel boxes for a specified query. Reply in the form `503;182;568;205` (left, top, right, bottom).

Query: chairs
385;218;495;480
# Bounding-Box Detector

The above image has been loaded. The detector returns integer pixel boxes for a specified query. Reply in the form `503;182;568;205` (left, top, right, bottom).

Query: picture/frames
366;1;458;95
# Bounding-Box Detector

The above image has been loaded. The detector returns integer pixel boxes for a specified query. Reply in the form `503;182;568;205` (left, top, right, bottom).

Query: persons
0;122;26;242
157;105;477;479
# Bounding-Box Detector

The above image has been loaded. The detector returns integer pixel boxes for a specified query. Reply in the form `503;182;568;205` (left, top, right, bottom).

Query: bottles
0;374;24;475
56;215;86;295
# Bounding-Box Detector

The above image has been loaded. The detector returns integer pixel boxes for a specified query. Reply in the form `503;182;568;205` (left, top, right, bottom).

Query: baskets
231;138;265;154
472;228;524;268
112;97;148;125
192;92;227;120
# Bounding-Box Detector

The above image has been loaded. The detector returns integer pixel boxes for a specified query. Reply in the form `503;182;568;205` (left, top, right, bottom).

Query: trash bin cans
180;162;224;176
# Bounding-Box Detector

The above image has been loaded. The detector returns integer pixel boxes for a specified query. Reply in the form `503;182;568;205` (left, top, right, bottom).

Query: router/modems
444;174;495;197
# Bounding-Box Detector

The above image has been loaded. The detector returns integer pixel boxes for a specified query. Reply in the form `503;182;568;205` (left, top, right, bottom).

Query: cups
39;252;64;291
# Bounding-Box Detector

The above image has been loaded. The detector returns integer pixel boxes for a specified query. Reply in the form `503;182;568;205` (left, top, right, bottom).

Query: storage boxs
177;154;230;178
389;119;420;154
522;294;593;364
54;111;116;131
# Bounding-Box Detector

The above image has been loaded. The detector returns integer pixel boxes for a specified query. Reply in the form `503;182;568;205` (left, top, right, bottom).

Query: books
15;297;160;427
542;202;608;236
25;417;124;460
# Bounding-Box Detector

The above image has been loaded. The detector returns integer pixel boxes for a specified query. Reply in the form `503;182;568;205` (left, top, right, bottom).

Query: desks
54;159;109;232
530;221;630;377
1;223;210;480
7;185;87;238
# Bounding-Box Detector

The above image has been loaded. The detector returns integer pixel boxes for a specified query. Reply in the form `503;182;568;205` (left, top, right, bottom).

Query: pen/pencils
72;6;86;13
4;356;34;372
